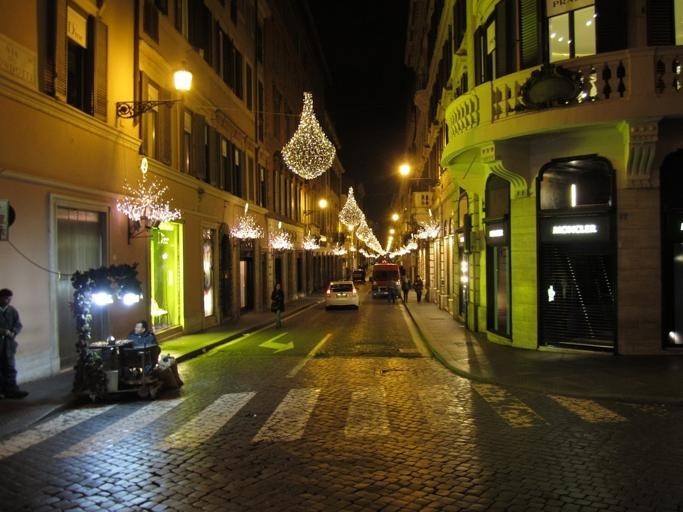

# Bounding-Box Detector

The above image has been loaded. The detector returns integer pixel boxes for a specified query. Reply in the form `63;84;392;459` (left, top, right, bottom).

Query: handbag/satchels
271;303;278;313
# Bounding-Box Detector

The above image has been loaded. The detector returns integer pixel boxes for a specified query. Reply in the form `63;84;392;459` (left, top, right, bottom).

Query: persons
412;274;422;302
269;282;285;330
126;319;161;377
402;276;410;303
387;278;396;304
0;287;28;399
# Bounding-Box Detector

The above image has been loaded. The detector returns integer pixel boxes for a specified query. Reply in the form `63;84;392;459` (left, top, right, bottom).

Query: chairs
150;298;169;329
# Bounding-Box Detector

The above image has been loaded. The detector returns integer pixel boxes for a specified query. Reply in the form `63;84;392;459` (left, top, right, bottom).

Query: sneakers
0;391;29;400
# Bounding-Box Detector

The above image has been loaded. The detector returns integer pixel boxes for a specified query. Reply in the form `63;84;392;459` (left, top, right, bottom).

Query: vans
372;262;402;298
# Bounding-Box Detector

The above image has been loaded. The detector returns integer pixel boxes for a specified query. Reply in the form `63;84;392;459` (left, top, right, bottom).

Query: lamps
117;70;193;119
414;209;441;241
268;221;295;252
228;203;265;243
389;238;418;259
303;230;320;250
333;241;356;256
355;223;386;259
281;93;336;179
338;186;366;232
116;158;183;245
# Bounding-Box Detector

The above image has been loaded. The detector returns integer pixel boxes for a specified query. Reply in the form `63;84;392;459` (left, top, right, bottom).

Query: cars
323;279;360;311
